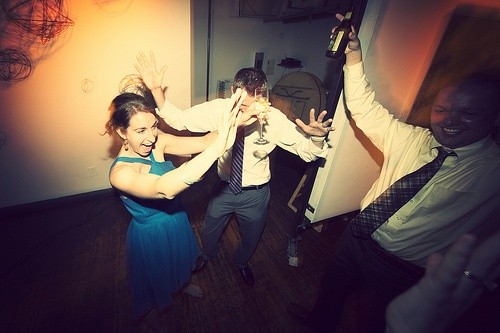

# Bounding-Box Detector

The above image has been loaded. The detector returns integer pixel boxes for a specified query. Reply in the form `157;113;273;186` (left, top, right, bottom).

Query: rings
463;270;478;281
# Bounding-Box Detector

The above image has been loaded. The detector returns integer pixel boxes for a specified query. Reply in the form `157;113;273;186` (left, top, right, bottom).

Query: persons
99;91;271;333
386;233;500;332
134;49;336;285
311;12;500;333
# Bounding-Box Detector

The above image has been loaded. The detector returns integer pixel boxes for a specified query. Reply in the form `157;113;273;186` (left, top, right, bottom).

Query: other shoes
287;302;310;327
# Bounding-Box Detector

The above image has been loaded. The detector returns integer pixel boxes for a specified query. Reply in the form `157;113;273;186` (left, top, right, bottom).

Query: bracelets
311;136;326;141
178;161;205;185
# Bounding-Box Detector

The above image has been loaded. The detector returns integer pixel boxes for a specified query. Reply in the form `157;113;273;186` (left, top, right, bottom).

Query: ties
347;146;457;240
229;127;245;195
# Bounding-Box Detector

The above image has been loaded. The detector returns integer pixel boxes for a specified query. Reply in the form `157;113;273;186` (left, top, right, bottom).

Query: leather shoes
193;256;208;272
238;265;255;286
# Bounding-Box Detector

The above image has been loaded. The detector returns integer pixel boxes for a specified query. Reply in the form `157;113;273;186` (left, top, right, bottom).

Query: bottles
325;3;359;60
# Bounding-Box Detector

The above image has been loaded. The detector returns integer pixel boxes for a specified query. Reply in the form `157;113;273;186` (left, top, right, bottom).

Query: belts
217;173;271;191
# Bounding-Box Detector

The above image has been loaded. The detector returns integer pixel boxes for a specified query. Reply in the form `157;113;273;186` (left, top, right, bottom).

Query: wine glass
255;87;270;146
